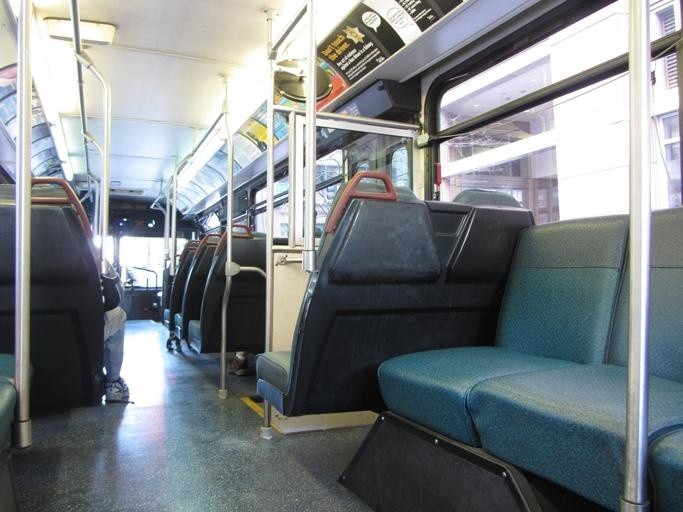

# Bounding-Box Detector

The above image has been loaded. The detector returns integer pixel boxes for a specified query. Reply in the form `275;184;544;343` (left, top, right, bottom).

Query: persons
99;273;129;401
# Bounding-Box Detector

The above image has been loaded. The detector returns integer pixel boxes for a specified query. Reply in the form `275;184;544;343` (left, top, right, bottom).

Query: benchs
0;174;110;508
160;172;683;508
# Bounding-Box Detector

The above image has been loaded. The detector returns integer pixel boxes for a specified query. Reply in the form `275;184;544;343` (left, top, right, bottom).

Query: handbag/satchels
101;273;123;311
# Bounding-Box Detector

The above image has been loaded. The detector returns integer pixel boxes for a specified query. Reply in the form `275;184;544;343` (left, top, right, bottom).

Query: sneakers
226;358;248;376
106;376;129;402
